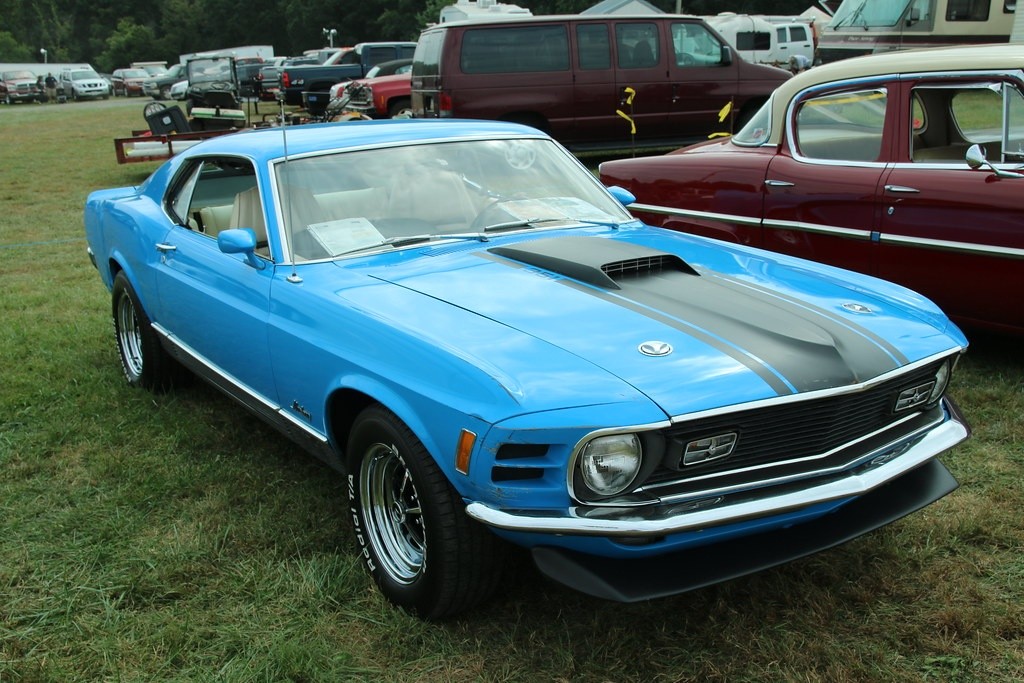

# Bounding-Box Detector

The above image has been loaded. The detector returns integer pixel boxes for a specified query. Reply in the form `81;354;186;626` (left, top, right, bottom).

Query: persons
44;72;58;103
34;75;44;104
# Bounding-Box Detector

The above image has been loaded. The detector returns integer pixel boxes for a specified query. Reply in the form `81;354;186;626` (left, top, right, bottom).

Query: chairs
388;165;478;230
618;37;655;67
231;185;324;255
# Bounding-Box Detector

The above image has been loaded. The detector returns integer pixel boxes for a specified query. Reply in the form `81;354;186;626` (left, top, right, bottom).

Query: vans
410;13;794;167
774;24;816;73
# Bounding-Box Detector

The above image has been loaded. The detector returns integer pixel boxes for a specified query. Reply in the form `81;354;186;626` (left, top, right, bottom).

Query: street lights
323;28;337;48
39;48;48;63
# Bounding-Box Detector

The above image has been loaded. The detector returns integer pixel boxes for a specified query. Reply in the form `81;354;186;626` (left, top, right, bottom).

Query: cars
364;58;412;78
169;47;343;117
598;42;1024;333
142;63;187;100
329;75;411;118
82;116;970;622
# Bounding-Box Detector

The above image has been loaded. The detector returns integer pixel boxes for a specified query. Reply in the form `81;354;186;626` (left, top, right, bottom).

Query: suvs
59;70;110;100
0;69;38;105
111;68;152;97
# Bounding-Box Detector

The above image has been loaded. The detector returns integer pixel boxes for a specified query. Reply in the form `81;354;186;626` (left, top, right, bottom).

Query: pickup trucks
258;41;417;115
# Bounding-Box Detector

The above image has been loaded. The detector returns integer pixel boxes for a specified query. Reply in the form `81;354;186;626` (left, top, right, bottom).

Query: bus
815;0;1018;65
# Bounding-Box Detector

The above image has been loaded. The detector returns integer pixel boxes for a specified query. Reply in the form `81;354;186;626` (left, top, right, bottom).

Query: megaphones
321;27;329;34
332;28;337;35
